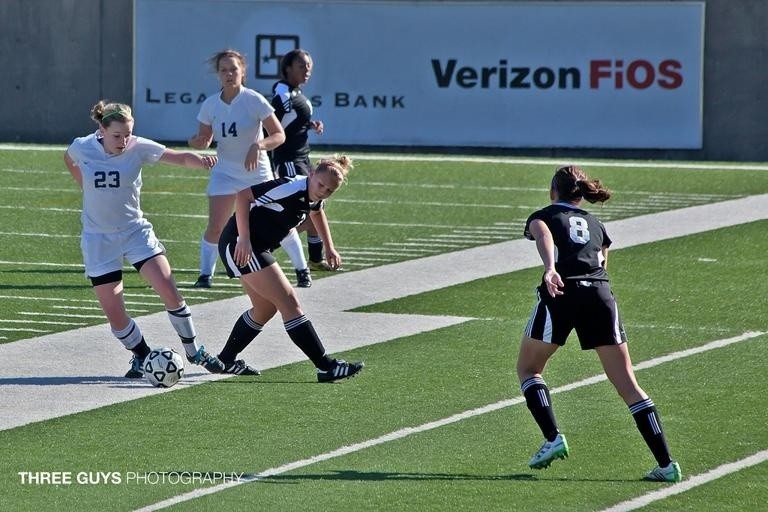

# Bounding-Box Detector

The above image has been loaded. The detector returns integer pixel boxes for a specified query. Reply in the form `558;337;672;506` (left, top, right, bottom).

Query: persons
190;49;313;289
517;165;683;484
258;50;340;272
215;154;365;385
63;100;226;379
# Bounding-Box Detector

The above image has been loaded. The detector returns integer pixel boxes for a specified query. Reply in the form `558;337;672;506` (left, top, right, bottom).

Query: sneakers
125;348;151;378
295;268;312;287
645;462;681;482
317;358;363;383
307;258;344;271
529;434;568;470
186;350;260;375
195;275;212;288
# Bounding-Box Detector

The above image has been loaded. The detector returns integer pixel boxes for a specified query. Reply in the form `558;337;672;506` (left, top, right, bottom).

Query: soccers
143;347;185;388
